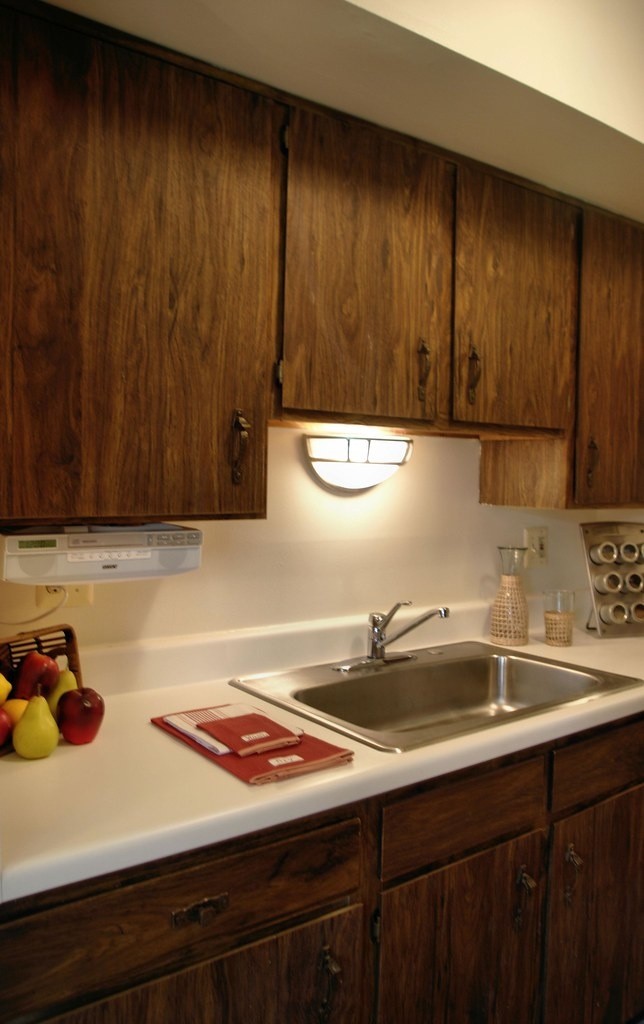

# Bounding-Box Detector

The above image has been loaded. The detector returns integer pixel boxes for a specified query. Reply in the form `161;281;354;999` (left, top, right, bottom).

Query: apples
57;688;105;744
0;707;12;747
12;650;60;700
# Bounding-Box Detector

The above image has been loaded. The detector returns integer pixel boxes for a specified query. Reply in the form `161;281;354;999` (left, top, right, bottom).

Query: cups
542;589;574;647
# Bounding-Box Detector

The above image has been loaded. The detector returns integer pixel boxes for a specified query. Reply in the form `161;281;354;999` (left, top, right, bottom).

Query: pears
48;667;79;721
12;684;58;758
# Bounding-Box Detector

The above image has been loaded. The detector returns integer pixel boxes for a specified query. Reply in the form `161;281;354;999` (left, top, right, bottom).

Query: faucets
367;600;450;660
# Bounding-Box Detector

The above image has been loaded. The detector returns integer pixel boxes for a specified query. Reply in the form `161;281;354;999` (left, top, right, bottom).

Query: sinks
229;639;644;753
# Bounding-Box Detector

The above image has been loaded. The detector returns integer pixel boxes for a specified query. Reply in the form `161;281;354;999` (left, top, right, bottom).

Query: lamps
302;433;414;496
35;585;94;608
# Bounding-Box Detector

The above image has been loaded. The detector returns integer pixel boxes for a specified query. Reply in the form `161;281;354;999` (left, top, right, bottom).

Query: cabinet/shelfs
0;4;281;524
275;104;572;440
1;817;368;1024
481;206;644;511
372;715;644;1024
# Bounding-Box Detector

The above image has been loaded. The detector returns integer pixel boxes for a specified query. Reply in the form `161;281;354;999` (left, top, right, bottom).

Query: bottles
489;546;532;646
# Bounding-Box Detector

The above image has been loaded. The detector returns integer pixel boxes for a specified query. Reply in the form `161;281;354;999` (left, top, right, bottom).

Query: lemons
0;673;28;724
